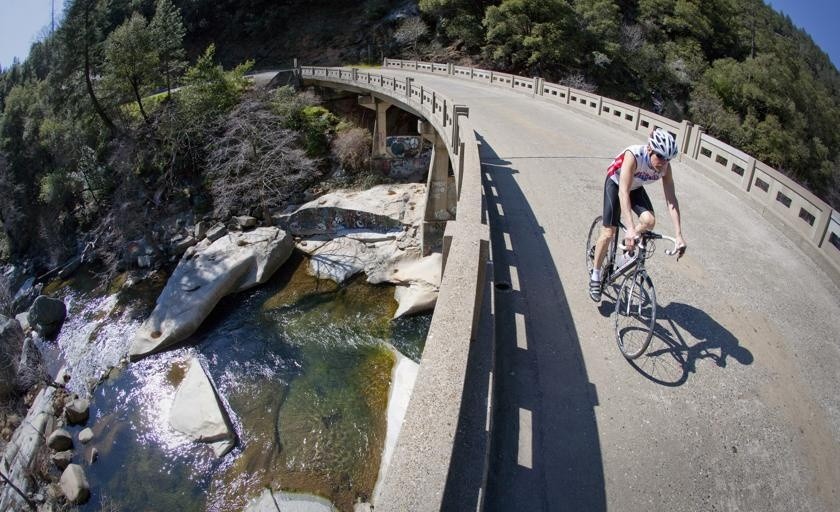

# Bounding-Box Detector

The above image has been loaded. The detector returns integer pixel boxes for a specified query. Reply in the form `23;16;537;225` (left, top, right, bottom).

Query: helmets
649;128;678;161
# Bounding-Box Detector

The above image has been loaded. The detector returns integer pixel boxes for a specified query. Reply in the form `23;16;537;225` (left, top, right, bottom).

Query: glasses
656;153;671;161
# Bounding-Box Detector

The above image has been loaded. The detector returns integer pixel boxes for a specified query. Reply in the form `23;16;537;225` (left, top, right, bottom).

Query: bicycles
585;217;680;359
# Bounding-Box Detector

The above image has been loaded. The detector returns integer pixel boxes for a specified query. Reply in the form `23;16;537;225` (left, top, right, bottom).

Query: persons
589;127;685;302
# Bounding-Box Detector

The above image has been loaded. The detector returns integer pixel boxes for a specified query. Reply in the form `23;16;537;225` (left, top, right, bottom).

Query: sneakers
589;278;602;302
623;251;637;264
